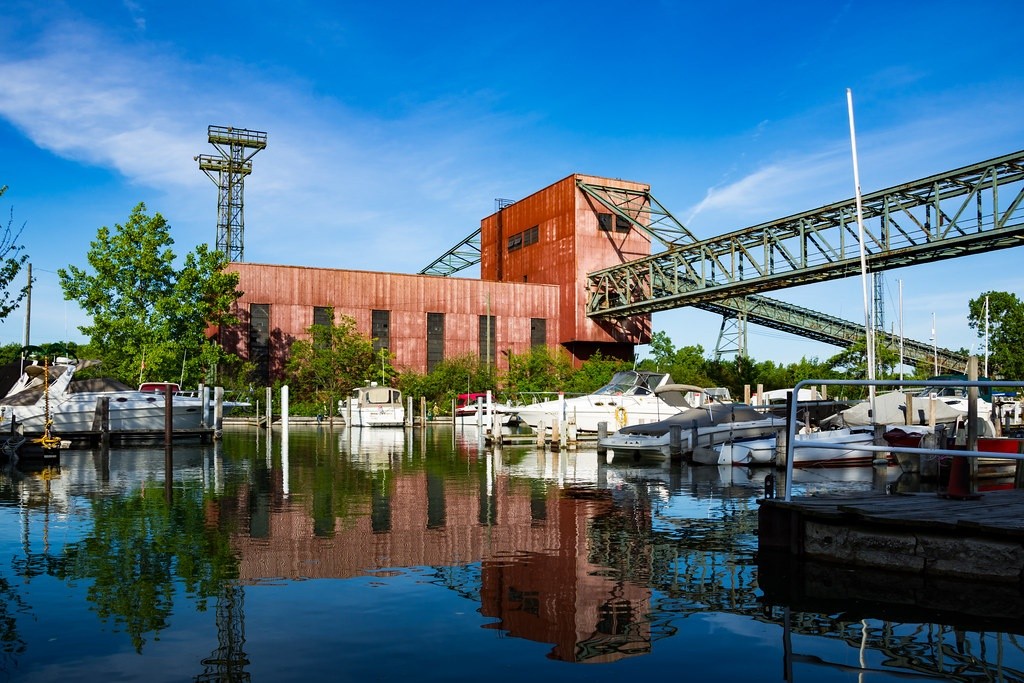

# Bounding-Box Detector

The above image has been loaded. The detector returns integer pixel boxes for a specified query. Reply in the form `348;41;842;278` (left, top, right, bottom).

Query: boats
338;427;405;496
337;348;404;427
517;89;1024;472
0;347;254;446
455;374;512;425
